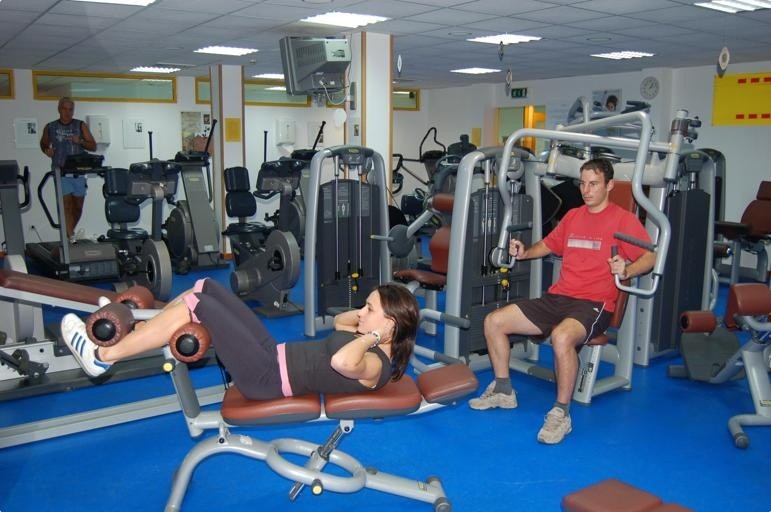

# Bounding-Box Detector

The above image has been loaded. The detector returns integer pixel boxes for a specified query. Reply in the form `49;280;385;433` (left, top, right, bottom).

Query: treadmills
26;153;122;283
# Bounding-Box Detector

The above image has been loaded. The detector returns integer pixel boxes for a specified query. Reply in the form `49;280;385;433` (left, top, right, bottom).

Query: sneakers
537;407;572;444
98;297;112;307
468;380;518;410
59;313;113;378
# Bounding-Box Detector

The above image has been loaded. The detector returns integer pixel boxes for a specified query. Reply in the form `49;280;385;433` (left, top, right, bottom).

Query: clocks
640;76;660;100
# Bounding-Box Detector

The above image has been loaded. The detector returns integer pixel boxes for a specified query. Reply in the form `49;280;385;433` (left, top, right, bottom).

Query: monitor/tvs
279;35;352;95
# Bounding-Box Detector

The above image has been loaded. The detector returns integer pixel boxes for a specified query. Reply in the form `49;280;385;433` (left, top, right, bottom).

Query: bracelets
366;331;380;350
78;140;88;148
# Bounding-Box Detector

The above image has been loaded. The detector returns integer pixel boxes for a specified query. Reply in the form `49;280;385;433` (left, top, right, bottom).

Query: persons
39;97;96;244
605;95;618;112
58;276;419;403
467;160;657;444
